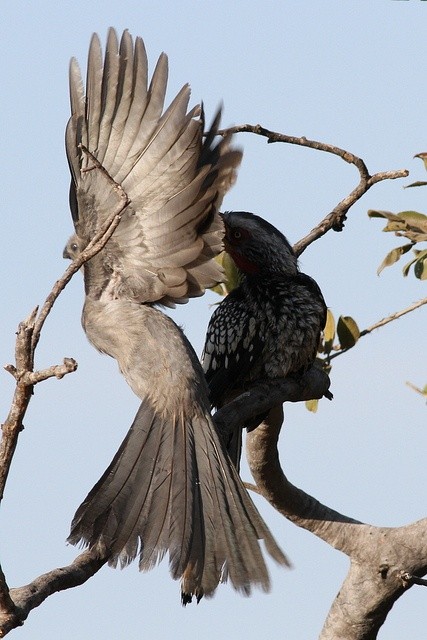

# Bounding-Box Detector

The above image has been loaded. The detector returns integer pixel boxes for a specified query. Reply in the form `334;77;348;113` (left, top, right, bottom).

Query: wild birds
201;211;328;436
62;26;292;606
